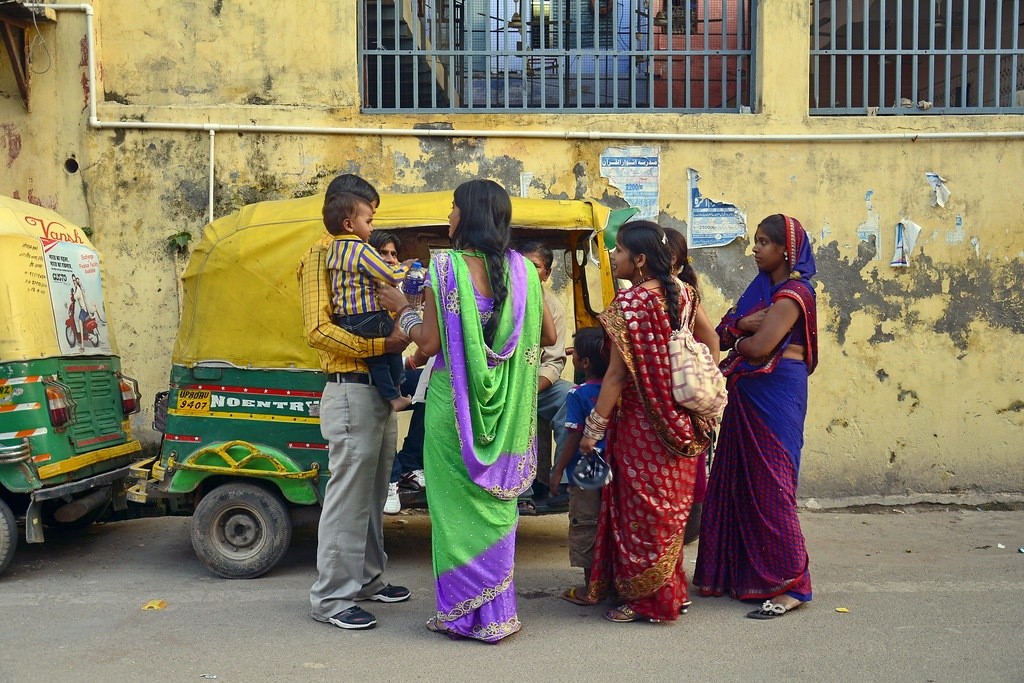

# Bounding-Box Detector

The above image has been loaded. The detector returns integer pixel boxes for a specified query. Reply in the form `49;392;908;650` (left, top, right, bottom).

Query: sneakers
382;483;401;513
354;581;411;602
323;604;376;629
397;469;426;492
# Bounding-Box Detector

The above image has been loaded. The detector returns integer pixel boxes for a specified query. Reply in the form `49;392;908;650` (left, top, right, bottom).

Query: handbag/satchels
668;276;728;415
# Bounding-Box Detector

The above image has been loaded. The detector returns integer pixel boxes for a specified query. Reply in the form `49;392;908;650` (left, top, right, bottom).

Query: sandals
427;616;461;638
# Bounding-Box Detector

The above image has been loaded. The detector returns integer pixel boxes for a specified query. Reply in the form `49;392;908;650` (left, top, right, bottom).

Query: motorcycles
128;186;643;581
0;195;144;576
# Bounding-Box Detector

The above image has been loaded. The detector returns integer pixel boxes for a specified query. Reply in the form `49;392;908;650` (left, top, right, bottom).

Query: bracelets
582;407;610;441
398;310;423;341
733;335;747;354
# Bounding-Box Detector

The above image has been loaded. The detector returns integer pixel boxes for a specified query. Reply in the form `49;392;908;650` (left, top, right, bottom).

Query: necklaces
632;274;658;288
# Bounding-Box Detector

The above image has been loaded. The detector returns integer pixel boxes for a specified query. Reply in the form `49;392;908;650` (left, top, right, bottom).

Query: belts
328;371;376;387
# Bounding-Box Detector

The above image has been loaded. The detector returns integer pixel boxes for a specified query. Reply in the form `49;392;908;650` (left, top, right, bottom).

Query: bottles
403;262;425;317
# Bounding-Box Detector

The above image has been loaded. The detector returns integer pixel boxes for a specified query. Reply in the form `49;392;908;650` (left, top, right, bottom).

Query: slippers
516;500;538;516
747;600;788;619
560;587;589;606
604;605;644;622
546;487;571;507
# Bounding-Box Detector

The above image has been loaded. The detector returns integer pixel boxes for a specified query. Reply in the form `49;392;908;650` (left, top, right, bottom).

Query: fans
810;17;846;38
623;0;722;29
478;0;573;35
890;0;980;42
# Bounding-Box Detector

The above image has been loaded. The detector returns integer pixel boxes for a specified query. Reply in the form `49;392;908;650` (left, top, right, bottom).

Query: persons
297;172;413;631
509;238;580;515
549;328;617;601
692;213;818;620
322;193;420;413
376;180;557;645
662;225;700;289
366;228;425;514
555;221;721;625
